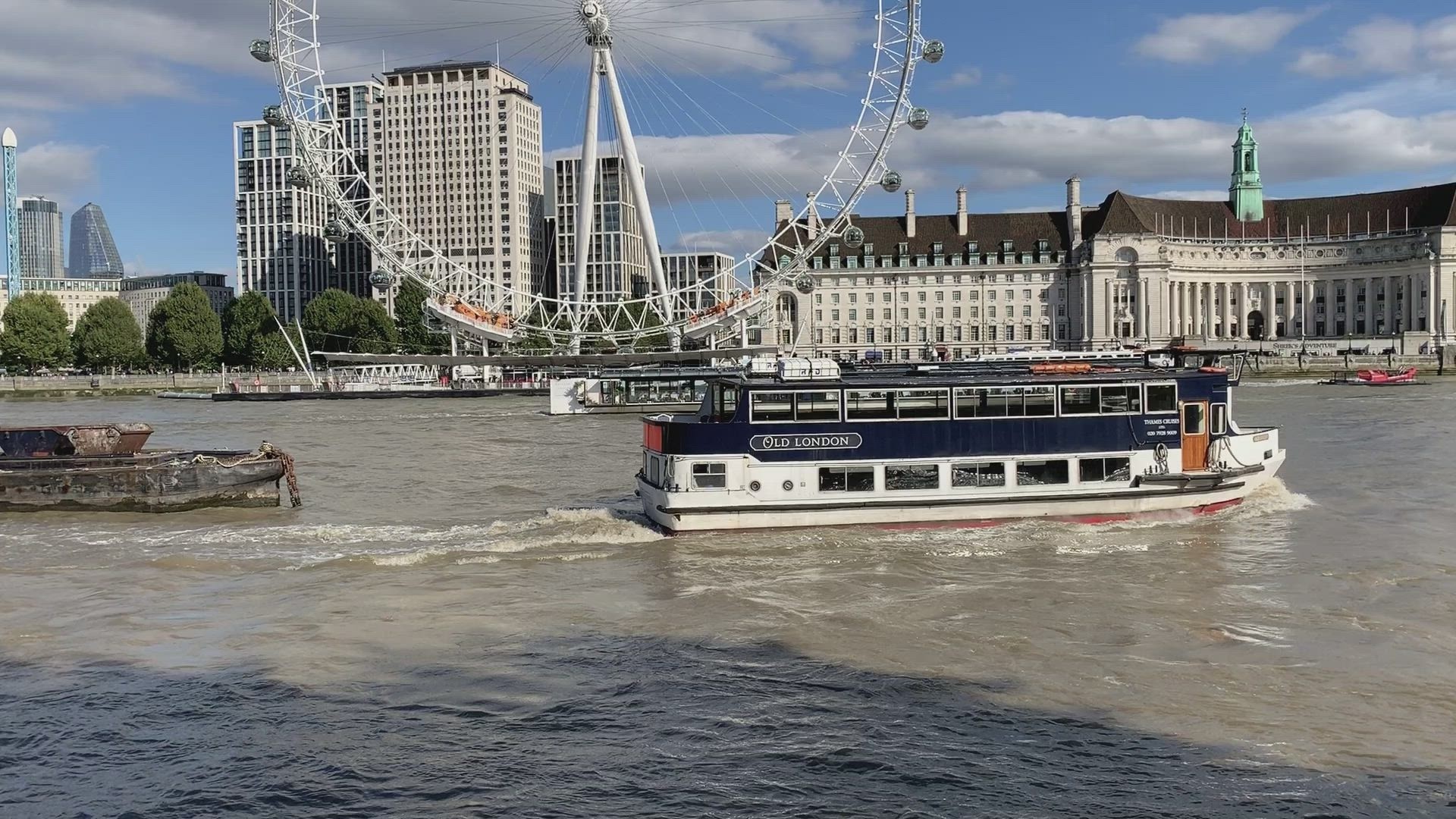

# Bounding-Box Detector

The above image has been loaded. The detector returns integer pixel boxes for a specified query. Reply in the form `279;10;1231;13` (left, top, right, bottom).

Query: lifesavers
1200;367;1226;373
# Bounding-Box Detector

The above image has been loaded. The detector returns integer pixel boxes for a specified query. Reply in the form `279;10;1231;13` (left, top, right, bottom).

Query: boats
1318;363;1432;386
633;336;1287;537
0;422;304;519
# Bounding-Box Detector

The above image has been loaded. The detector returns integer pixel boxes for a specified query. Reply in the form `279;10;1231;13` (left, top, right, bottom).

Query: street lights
1258;333;1265;356
1390;331;1396;353
1347;332;1354;354
873;341;877;363
1300;334;1308;355
